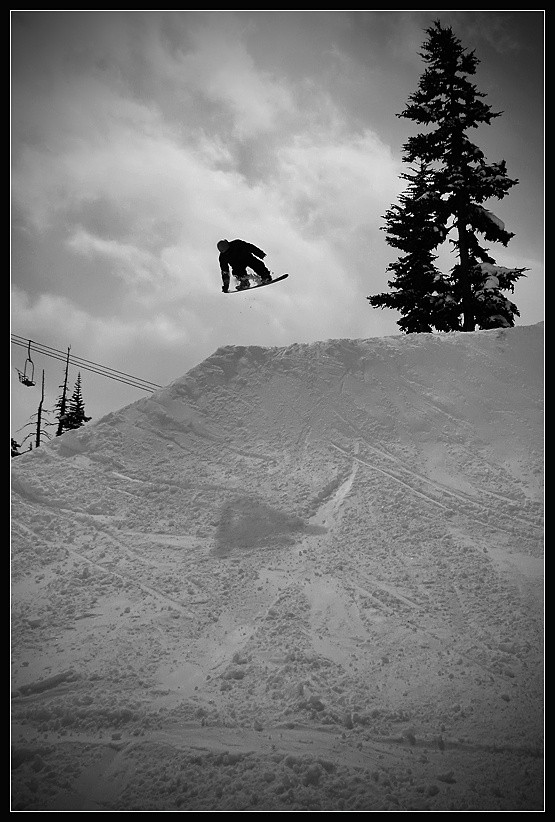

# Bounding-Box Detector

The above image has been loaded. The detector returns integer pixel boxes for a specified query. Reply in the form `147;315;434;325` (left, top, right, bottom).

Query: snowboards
224;273;289;293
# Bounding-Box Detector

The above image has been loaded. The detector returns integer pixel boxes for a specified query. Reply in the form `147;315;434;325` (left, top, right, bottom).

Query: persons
217;238;273;293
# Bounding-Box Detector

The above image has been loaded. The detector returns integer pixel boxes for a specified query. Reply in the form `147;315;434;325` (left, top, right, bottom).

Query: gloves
222;284;229;293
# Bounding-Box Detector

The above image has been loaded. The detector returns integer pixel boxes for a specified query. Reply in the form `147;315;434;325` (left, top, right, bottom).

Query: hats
216;241;228;251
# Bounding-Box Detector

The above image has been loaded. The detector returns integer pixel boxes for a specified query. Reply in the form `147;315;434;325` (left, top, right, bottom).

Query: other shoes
236;281;250;290
261;275;272;284
